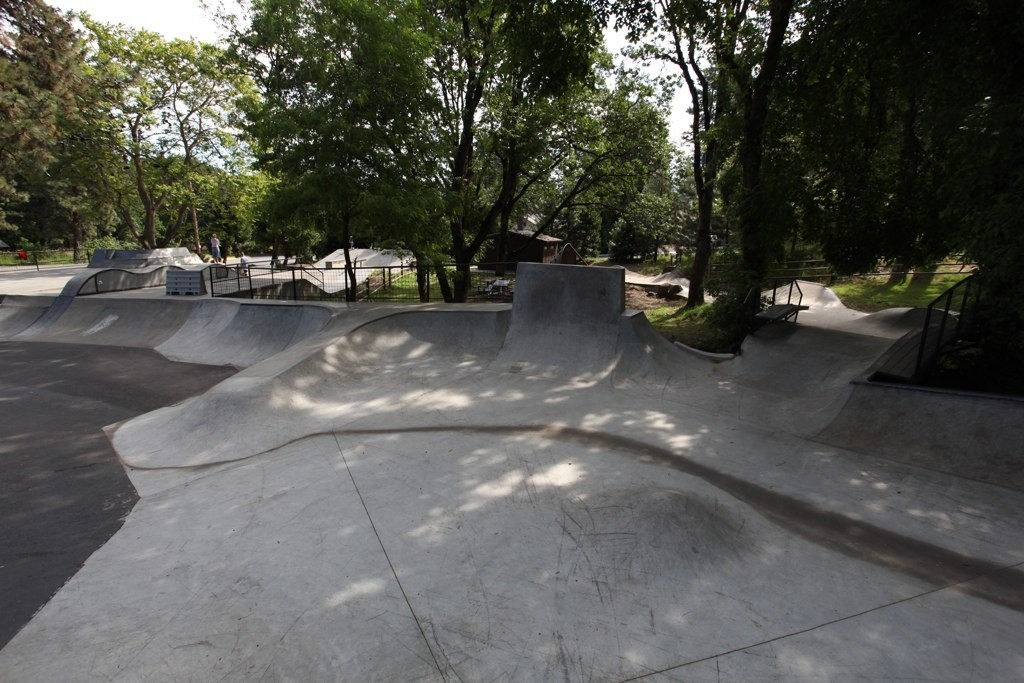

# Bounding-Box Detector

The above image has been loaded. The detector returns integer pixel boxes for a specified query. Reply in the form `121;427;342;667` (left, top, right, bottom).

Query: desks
480;276;504;287
494;279;510;296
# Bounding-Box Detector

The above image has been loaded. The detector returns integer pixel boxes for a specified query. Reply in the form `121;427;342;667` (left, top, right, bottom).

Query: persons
240;252;248;275
211;234;220;263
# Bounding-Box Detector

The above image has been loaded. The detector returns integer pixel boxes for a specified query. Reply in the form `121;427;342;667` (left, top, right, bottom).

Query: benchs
756;304;809;324
